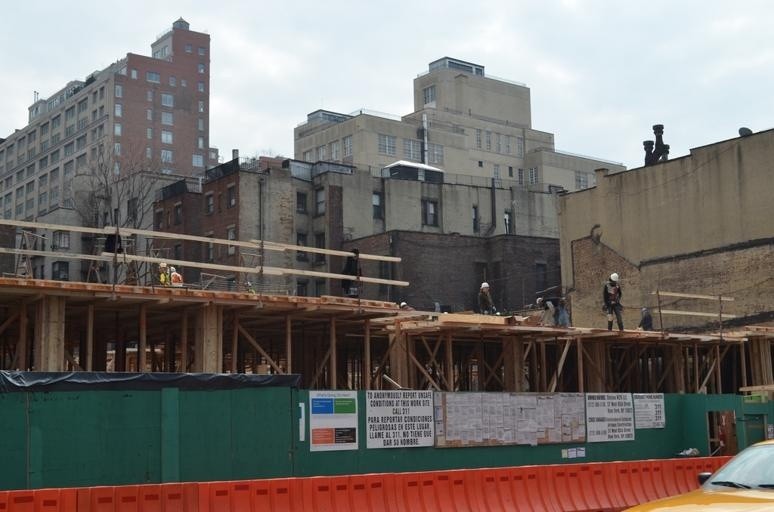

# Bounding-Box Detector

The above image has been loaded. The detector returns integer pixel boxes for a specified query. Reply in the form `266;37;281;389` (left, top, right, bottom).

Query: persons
601;272;624;330
636;307;652;331
477;282;496;315
536;296;569;328
170;267;182;284
400;302;416;311
496;307;512;316
341;247;364;298
159;262;171;286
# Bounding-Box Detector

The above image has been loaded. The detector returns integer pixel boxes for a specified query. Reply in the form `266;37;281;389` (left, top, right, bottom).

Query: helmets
536;298;543;304
610;273;618;281
481;282;489;288
159;262;176;272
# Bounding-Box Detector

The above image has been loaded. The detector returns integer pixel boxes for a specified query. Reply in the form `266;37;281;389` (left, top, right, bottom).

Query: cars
620;440;774;512
